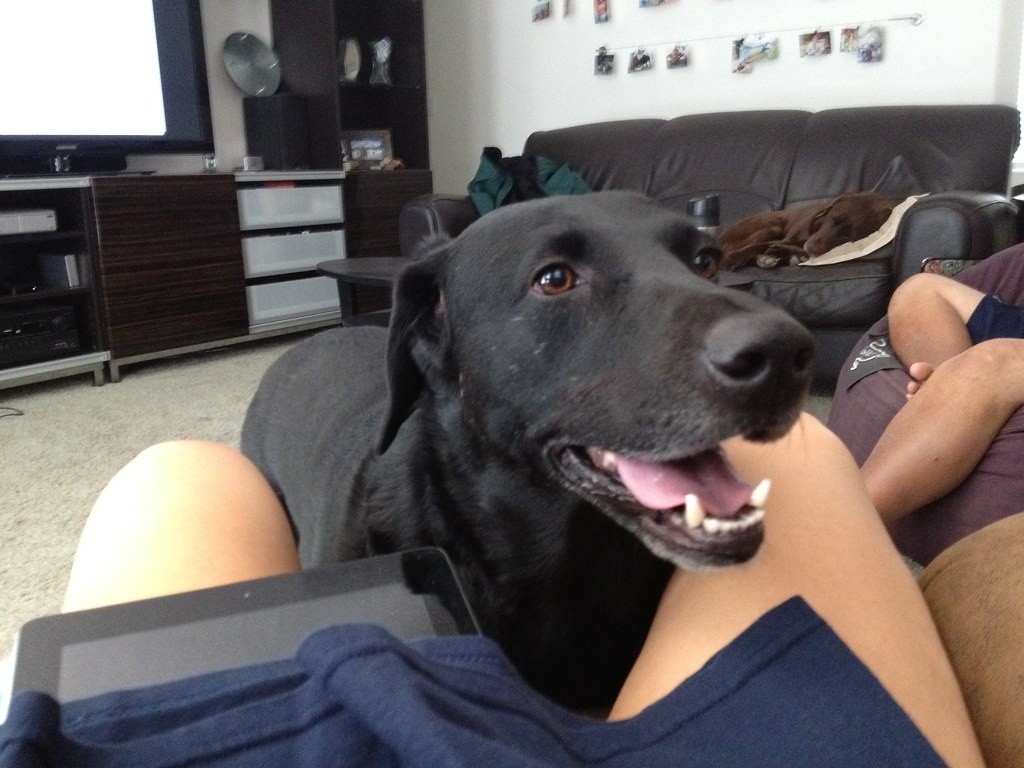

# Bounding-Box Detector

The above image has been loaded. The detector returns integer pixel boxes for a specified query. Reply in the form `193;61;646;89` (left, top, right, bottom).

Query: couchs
394;102;1022;389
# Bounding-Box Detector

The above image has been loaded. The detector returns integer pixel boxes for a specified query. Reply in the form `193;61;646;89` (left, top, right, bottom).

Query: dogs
235;186;816;713
714;191;894;273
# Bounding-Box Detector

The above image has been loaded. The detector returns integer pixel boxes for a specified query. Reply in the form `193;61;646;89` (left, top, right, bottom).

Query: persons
853;265;1023;524
0;400;991;768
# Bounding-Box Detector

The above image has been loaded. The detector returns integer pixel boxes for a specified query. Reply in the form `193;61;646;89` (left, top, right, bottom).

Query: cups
201;155;217;172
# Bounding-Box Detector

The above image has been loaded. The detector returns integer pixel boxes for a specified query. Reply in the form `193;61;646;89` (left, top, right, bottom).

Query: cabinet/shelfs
0;0;436;394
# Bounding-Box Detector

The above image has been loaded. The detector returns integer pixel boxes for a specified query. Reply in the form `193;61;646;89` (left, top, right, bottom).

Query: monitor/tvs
0;0;214;155
